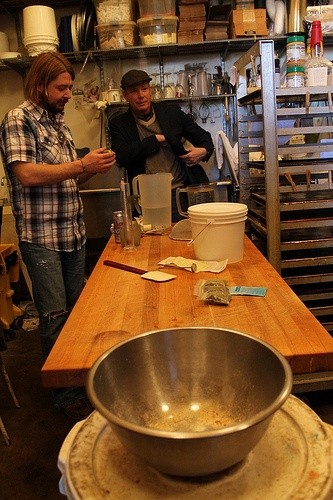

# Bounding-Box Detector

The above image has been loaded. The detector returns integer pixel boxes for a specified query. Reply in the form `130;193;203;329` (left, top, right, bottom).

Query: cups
107;89;120;102
191;71;211;97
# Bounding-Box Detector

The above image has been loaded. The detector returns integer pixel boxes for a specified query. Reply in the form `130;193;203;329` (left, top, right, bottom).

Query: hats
120;69;152;89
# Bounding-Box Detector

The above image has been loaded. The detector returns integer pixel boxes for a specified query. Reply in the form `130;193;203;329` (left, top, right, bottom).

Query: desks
41;223;333;388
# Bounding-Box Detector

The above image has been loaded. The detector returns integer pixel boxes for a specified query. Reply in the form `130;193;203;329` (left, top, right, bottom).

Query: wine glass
151;71;195;100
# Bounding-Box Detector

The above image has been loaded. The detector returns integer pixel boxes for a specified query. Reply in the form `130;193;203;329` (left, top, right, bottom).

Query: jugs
132;172;174;231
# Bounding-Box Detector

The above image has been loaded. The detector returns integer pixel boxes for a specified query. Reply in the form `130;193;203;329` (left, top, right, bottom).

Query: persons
1;49;117;420
108;69;215;218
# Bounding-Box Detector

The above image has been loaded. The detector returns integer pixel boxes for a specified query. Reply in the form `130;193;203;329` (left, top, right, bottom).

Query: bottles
304;20;333;87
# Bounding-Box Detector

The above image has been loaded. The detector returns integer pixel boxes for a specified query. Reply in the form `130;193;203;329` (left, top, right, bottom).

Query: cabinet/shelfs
233;40;333;337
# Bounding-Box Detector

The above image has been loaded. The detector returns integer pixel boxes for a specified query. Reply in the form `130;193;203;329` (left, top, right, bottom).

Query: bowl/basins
85;327;293;477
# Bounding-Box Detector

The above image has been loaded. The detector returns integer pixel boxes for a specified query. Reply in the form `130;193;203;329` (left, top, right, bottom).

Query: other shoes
55;393;89;421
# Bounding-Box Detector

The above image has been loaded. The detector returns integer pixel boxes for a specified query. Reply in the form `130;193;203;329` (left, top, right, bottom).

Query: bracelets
78;158;86;173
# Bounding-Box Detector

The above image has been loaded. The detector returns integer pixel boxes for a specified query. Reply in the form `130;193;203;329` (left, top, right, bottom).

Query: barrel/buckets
23;6;59;56
58;394;333;500
187;202;248;264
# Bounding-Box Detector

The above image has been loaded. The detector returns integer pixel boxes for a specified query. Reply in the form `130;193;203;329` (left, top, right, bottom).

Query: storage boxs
93;0;178;50
229;9;267;39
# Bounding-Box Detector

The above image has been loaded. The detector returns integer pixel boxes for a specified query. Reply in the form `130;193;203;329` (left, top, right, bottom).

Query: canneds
286;36;306;87
114;210;125;243
